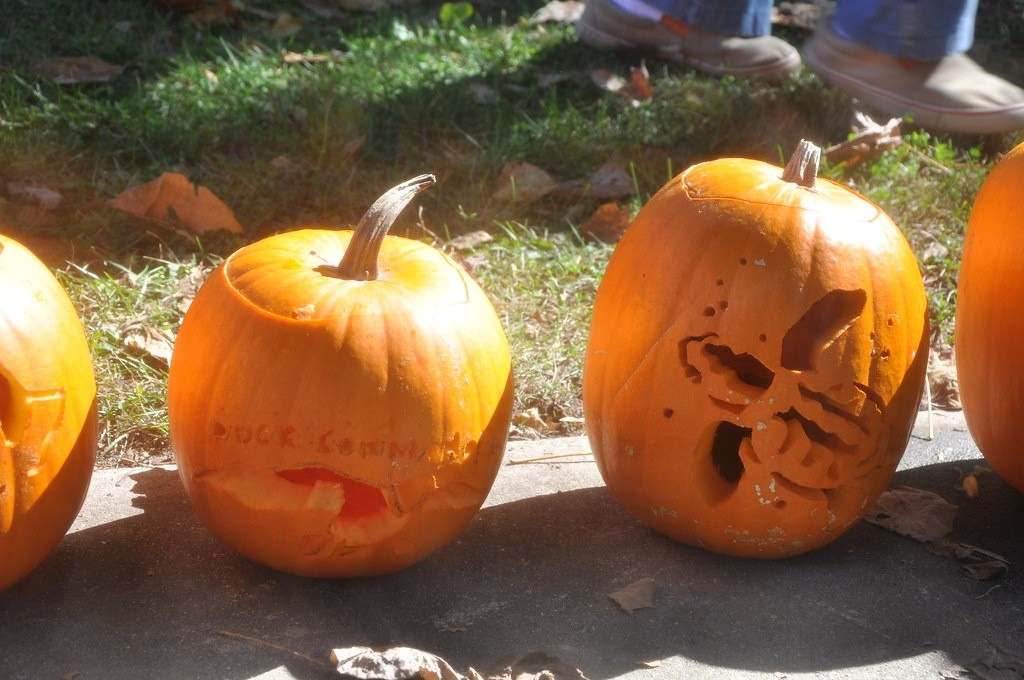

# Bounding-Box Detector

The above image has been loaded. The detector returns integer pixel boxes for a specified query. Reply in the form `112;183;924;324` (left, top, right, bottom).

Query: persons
573;0;1023;134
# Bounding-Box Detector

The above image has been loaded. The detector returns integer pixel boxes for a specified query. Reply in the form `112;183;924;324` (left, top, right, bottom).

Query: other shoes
803;14;1024;135
574;0;801;83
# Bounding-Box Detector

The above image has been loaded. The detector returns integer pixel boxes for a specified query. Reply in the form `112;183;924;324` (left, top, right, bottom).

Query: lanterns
1;139;1024;593
